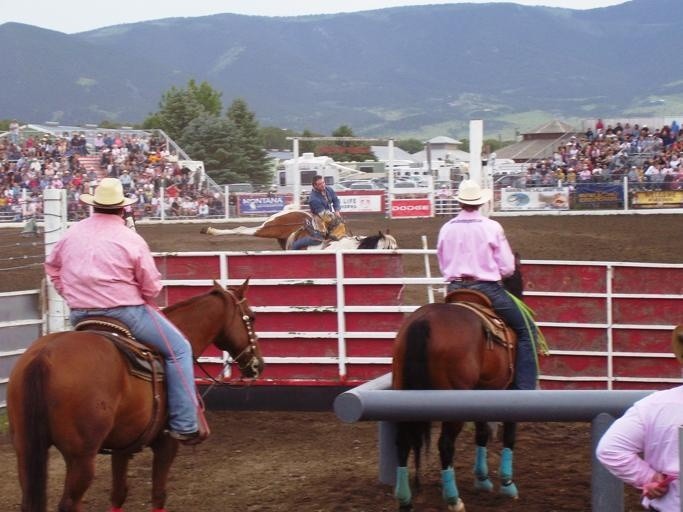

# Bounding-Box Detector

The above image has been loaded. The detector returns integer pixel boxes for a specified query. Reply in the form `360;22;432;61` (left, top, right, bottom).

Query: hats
441;180;495;207
73;175;140;213
669;322;682;369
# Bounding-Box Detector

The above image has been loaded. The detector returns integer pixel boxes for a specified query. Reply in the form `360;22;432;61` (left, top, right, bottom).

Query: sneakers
168;430;201;441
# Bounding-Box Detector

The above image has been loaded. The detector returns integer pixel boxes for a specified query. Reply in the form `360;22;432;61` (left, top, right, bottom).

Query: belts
71;306;110;313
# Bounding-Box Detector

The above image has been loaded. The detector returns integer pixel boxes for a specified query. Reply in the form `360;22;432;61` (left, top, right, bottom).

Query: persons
497;123;682;186
0;132;235;220
435;178;539;391
289;176;341;252
594;383;683;512
41;177;207;442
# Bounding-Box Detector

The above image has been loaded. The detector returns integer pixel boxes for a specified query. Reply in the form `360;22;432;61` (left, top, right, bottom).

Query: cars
336;159;461;190
489;170;528;189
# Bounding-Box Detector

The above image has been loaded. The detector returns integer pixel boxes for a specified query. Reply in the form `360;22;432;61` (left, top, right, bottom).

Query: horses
200;204;399;250
393;252;523;512
7;275;264;512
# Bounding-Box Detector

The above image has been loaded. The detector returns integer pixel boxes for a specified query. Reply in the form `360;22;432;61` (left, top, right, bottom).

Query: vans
210;183;253;205
